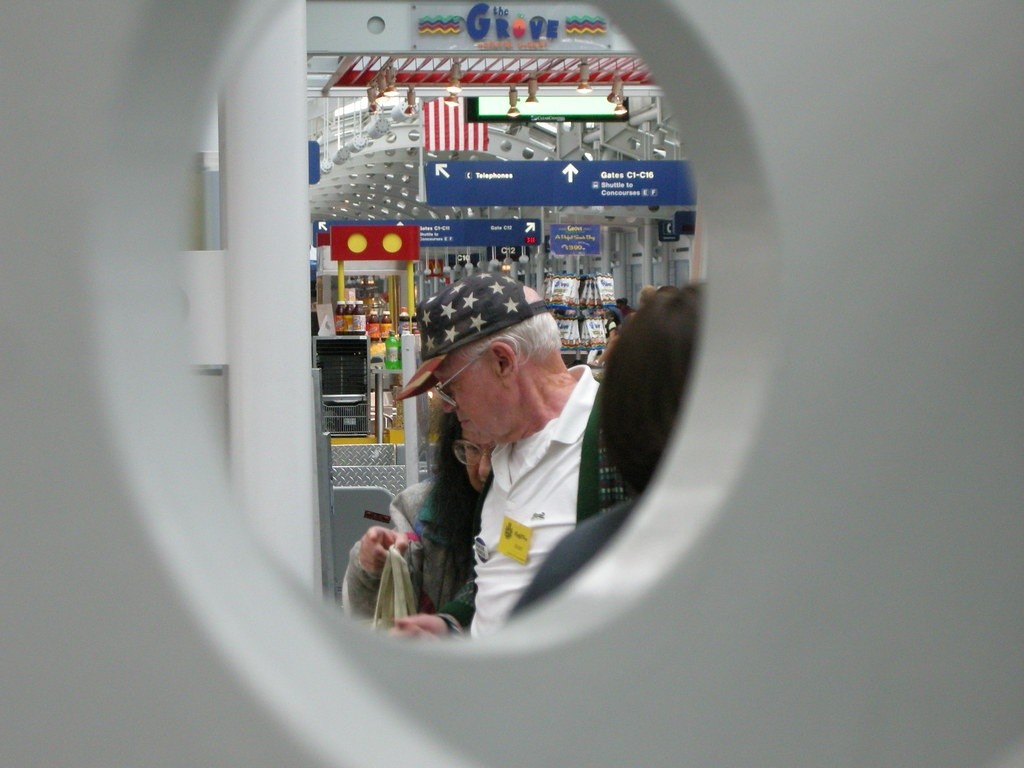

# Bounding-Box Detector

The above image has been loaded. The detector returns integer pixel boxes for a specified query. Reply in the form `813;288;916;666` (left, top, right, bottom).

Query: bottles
397;306;410;337
369;306;381;342
380;310;393;342
335;300;366;335
385;329;400;370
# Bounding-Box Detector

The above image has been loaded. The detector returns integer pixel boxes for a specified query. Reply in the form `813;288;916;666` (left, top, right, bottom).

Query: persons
386;275;626;640
510;283;701;620
342;410;494;624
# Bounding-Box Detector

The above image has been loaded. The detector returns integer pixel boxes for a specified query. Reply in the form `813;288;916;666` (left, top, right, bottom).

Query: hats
394;272;547;401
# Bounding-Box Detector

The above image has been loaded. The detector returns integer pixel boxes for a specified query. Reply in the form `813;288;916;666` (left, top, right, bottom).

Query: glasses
449;438;493;466
433;357;481;408
